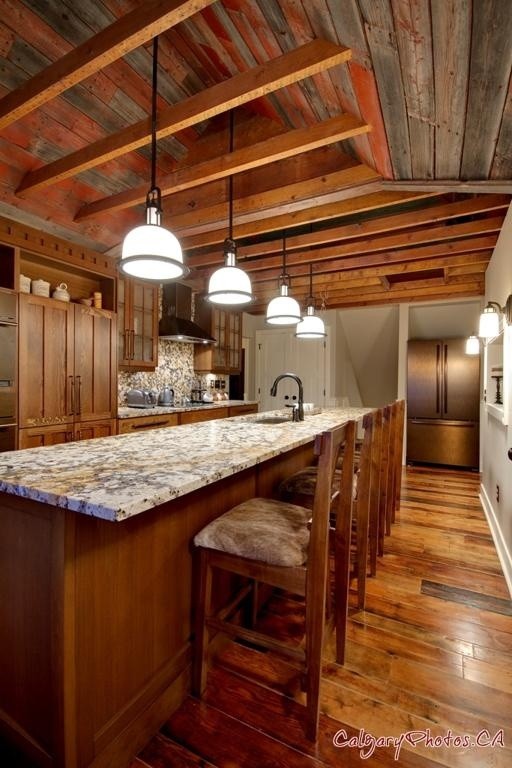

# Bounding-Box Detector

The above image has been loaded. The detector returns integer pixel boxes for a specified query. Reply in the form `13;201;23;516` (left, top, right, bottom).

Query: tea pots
158;384;175;406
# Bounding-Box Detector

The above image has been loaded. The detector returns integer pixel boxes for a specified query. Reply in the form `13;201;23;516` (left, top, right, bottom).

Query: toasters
126;387;157;409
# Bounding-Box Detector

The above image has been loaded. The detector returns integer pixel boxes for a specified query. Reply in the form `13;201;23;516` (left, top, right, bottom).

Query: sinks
255;417;293;424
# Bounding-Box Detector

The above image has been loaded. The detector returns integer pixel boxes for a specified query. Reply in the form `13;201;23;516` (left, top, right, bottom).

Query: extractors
160;282;216;346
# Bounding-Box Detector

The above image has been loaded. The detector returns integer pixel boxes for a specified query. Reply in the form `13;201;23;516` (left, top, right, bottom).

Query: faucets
270;374;303;419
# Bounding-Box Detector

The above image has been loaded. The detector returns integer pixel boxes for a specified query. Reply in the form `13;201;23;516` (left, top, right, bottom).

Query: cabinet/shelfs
117;276;159;372
18;419;117;450
118;413;178;434
195;294;243;375
18;293;117;429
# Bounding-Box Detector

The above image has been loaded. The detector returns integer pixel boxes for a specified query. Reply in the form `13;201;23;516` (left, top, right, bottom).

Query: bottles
94;290;103;309
20;273;70;303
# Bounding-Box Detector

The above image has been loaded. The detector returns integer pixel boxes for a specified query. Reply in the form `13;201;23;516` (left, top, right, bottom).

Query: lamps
204;108;257;308
118;36;191;284
464;332;485;355
294;262;327;339
478;294;512;337
264;238;304;325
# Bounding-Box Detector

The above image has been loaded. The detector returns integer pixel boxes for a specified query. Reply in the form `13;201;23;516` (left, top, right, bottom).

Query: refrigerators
406;338;481;471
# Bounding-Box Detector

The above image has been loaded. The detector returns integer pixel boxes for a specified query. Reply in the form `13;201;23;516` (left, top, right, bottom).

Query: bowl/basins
80;298;93;309
